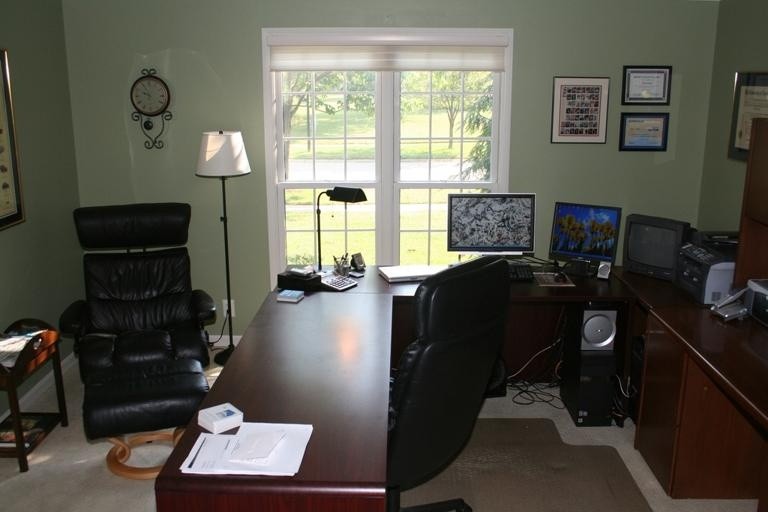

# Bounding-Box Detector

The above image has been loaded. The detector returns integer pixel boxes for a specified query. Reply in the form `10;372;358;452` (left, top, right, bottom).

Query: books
378;262;434;283
0;415;45;449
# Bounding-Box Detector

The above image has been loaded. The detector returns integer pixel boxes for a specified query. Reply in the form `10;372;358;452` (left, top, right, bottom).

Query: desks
613;267;768;511
153;265;637;511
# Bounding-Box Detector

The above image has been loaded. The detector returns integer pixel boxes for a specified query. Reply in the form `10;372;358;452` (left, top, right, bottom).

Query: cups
334;257;350;278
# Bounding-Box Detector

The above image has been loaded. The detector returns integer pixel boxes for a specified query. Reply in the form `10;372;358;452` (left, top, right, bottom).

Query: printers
673;242;736;304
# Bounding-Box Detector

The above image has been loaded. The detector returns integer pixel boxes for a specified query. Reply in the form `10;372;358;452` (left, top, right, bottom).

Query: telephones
710;288;748;323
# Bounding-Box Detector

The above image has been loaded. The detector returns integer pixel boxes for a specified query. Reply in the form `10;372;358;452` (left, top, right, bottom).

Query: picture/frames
618;112;668;152
727;72;768;162
550;76;610;145
621;65;672;106
0;50;26;233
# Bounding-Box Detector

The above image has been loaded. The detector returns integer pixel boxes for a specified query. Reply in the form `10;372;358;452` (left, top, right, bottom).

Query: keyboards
509;265;535;282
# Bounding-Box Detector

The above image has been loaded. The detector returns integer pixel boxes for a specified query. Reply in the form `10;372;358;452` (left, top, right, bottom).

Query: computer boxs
560;322;626;427
628;336;645;422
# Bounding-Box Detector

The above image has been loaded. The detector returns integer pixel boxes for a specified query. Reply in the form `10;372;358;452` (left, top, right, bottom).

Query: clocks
130;68;173;151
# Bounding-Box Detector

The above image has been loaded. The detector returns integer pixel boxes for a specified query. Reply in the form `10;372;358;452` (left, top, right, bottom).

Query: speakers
580;310;617;351
597;261;612;280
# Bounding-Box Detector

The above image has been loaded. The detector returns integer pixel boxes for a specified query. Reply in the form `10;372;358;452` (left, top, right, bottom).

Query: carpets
400;417;655;511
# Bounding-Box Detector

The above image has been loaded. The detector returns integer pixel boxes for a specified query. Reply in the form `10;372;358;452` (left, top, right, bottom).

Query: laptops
378;265;449;283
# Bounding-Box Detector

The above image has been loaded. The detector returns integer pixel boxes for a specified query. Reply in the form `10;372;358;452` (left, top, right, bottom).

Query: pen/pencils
333;252;348;274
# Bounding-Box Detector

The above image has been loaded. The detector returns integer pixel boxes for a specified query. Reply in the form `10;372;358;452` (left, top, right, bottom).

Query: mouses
554;272;567;282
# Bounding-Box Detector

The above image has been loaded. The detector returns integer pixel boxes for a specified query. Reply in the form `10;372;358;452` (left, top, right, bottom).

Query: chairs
386;253;515;511
57;202;219;483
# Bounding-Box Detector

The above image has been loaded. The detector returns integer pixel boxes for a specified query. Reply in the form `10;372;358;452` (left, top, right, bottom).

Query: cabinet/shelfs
0;317;70;473
731;116;768;294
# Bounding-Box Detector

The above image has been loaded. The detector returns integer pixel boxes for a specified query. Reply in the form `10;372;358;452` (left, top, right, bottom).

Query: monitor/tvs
623;214;697;288
549;201;622;263
447;193;536;253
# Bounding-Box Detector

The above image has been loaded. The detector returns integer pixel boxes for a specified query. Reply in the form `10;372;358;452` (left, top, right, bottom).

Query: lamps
195;130;253;367
316;186;368;272
